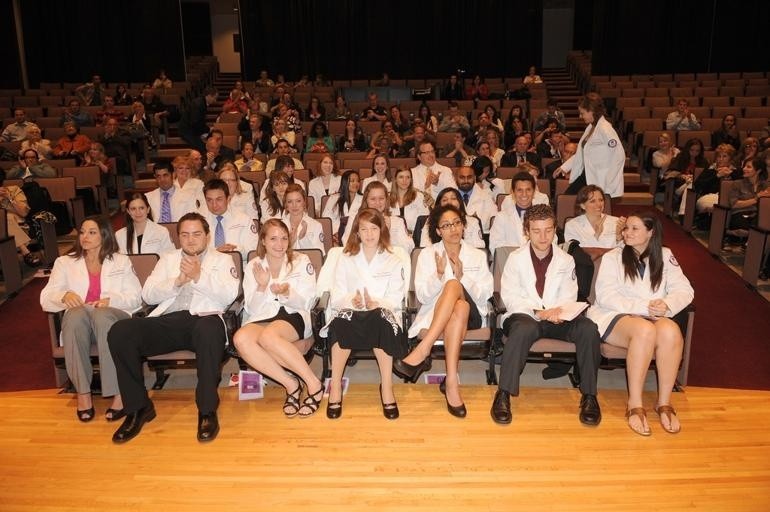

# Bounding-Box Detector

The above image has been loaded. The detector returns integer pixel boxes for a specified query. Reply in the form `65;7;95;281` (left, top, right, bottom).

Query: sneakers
23;252;41;267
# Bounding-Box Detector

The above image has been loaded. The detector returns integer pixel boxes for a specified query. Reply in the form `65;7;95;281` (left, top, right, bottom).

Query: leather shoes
578;393;603;426
490;388;514;426
326;383;346;419
195;402;222;442
111;400;158;445
379;383;400;419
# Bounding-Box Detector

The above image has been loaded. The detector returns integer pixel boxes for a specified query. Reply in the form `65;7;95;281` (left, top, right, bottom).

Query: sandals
282;376;304;418
104;400;127;421
298;381;325;415
74;390;97;423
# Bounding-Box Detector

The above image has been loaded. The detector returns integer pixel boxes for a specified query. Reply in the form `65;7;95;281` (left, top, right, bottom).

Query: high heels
438;375;469;418
393;353;434;384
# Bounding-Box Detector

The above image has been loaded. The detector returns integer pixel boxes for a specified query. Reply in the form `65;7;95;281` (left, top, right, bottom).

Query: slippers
624;401;654;436
655;401;683;433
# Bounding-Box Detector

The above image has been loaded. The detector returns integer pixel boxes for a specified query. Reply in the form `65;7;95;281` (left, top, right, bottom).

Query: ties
426;168;433;196
159;192;174;223
462;194;470;206
520;210;526;224
214;216;227;250
519;156;525;164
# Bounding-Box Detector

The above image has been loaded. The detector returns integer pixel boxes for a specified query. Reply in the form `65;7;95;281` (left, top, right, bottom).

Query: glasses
437;220;463;230
178;233;207;239
420;149;437;155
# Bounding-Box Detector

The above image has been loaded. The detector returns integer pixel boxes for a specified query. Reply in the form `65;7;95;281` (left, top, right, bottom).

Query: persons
652;98;770;281
1;66;695;442
